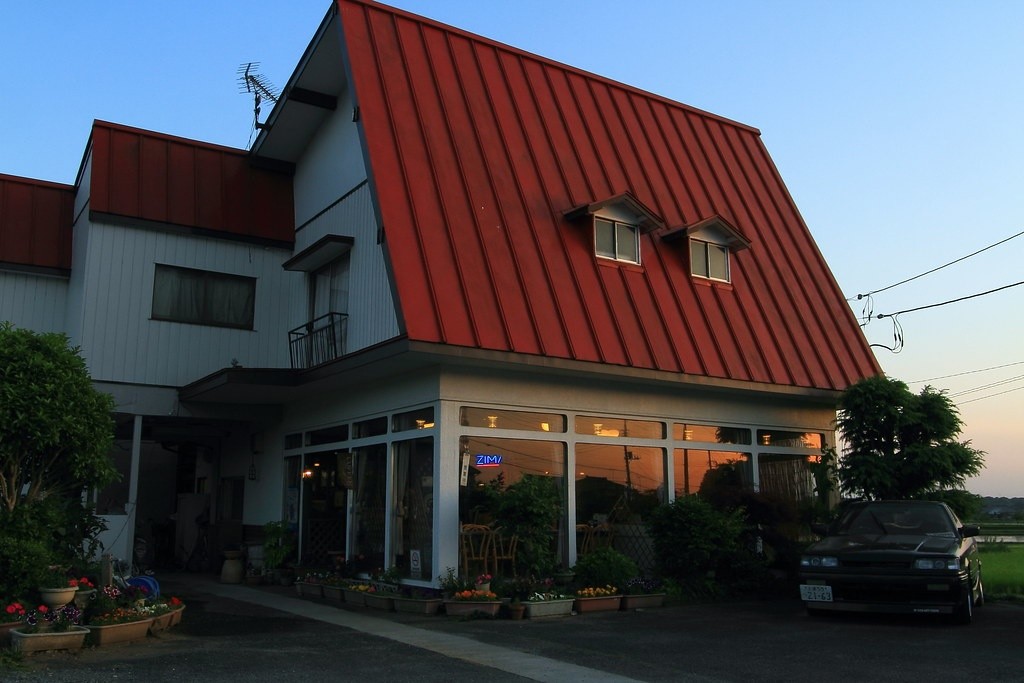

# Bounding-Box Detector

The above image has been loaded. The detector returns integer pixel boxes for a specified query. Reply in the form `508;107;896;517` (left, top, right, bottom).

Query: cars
796;498;986;627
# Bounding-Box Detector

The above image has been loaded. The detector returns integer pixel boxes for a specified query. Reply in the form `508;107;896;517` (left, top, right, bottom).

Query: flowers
6;576;181;632
295;556;402;593
528;577;617;602
626;576;657;595
454;575;496;602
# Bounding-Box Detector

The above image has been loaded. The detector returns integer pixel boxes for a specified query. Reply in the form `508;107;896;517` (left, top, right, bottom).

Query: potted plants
509;592;526;620
390;589;441;616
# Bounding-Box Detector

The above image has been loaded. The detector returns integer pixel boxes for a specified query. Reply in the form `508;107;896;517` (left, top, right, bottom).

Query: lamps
247;462;257;480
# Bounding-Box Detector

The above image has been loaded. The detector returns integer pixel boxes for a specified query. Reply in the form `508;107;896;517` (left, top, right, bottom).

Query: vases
474;580;491;591
294;582;394;611
0;602;185;652
520;599;575;620
574;596;622;612
623;594;666;609
443;599;503;617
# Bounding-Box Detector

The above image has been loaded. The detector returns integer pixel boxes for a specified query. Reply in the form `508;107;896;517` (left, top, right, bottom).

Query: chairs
458;521;615;581
